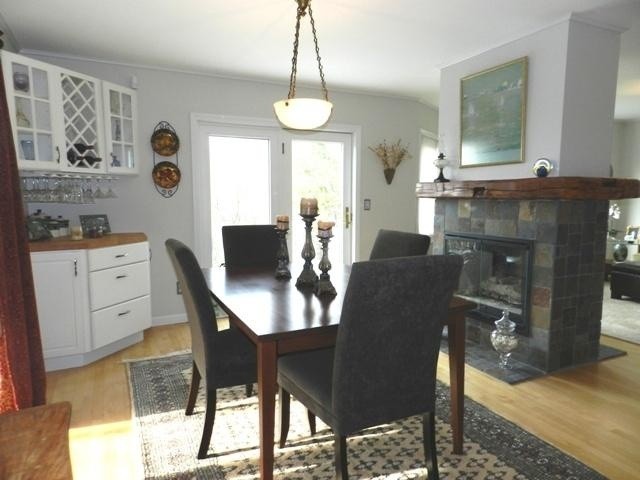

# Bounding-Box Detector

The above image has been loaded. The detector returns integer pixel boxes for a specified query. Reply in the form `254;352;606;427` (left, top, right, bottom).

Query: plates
149;128;180;157
151;160;182;189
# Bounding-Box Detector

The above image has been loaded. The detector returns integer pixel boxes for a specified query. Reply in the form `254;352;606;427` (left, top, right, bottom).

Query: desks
199;265;478;479
605;261;640;304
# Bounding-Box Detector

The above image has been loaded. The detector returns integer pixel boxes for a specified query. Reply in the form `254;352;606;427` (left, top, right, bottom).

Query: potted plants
368;137;412;185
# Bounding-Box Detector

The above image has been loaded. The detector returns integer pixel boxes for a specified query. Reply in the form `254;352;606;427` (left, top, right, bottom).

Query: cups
70;225;83;242
19;139;36;161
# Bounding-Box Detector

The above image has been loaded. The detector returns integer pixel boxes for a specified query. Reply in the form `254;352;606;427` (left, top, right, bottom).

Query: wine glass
19;172;119;206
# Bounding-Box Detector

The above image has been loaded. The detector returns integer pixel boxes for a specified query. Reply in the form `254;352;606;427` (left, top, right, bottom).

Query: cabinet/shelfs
1;50;62;171
85;242;153;350
26;249;87;357
58;65;107;175
100;79;141;175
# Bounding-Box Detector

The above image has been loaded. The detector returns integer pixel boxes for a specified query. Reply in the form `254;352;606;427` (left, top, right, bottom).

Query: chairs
164;238;318;459
221;224;289;268
276;254;463;478
369;229;431;260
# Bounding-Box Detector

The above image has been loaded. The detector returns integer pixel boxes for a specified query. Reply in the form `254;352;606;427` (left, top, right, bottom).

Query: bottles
13;70;30;93
55;142;103;169
27;208;69;239
15;102;31;129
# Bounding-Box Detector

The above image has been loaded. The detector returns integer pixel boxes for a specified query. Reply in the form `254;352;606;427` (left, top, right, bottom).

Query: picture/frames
626;225;640;244
461;56;528;167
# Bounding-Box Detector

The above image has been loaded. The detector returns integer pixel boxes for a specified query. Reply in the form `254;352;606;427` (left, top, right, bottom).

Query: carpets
124;344;609;480
599;280;640;346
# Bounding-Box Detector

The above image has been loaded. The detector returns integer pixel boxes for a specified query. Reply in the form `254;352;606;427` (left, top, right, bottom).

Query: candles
277;198;334;231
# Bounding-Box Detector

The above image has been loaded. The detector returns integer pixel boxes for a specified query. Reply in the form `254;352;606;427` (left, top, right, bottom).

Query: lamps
433;152;449;182
273;0;335;132
